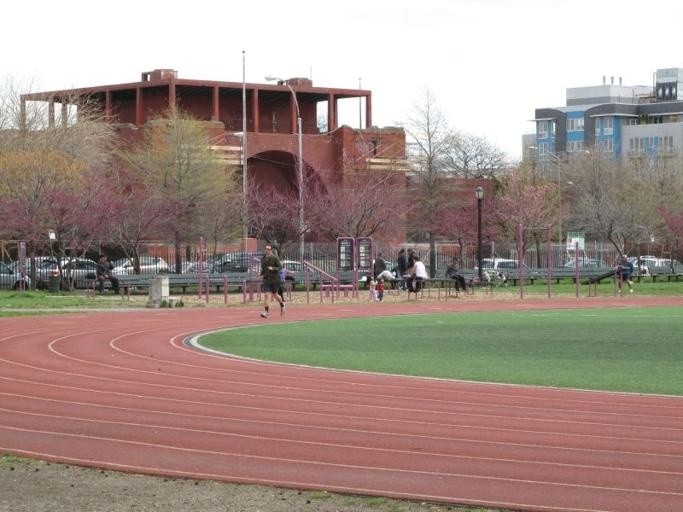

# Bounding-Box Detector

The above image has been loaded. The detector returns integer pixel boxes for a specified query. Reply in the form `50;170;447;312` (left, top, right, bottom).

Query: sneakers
261;311;269;318
281;303;285;310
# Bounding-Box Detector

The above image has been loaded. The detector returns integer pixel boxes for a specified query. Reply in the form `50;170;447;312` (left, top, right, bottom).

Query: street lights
265;74;307;275
474;185;486;285
526;144;565;267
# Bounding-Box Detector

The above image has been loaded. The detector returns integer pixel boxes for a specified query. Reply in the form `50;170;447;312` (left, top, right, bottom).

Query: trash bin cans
149;275;169;304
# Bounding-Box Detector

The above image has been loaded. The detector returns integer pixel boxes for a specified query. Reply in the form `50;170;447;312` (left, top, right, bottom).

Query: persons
618;254;633;293
637;260;649;276
97;255;120;294
366;248;427;303
260;244;286;318
445;257;467;293
